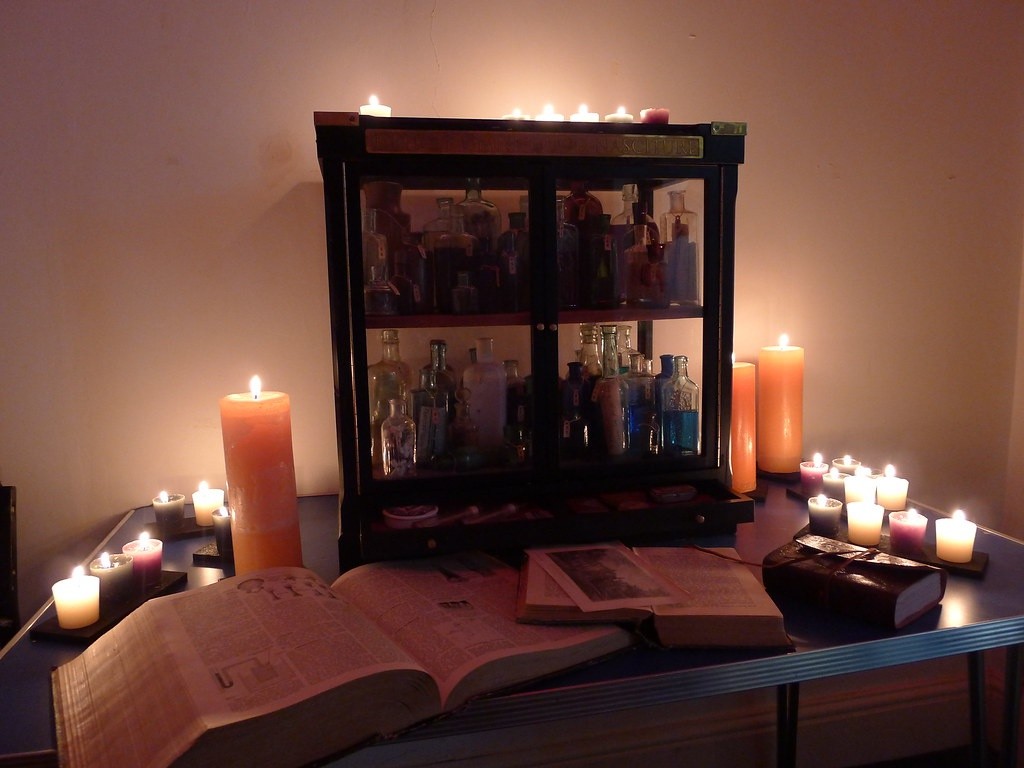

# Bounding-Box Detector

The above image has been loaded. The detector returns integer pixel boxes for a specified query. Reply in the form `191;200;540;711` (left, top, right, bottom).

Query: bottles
366;323;701;479
361;174;700;317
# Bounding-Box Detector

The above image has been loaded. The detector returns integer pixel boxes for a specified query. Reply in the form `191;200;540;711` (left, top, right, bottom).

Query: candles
90;552;136;599
799;453;909;509
152;491;187;531
757;334;804;474
888;506;929;553
360;94;391;118
807;492;843;533
221;373;303;577
52;565;100;630
209;504;235;578
732;352;757;492
845;499;883;547
935;510;977;563
503;106;670;124
192;481;225;525
123;530;163;585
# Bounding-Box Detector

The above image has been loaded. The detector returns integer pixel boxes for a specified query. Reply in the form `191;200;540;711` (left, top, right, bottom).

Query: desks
0;494;1024;768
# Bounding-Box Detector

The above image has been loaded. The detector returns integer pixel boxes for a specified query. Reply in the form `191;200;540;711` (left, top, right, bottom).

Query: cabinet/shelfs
312;110;747;569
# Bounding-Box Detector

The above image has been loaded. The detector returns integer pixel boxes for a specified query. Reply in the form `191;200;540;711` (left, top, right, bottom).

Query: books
519;541;796;650
50;550;632;768
